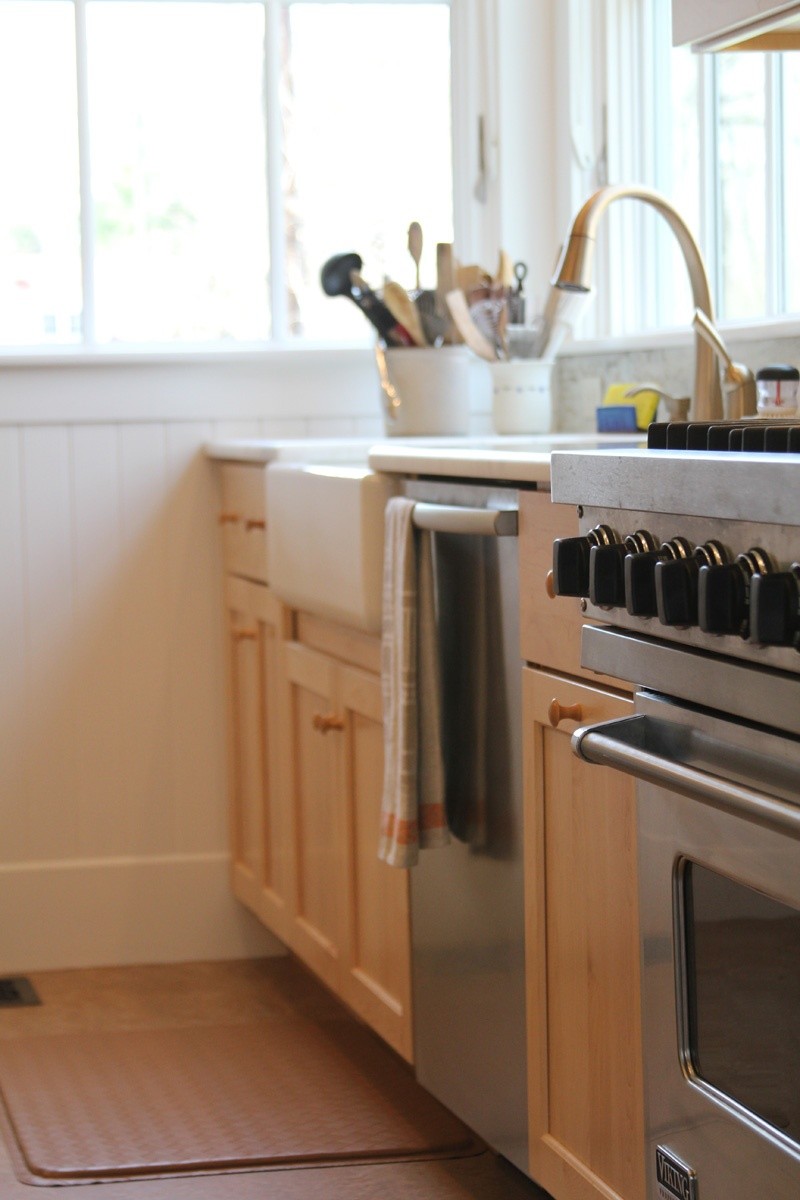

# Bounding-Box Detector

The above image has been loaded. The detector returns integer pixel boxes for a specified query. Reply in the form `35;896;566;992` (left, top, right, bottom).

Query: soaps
594;404;640;434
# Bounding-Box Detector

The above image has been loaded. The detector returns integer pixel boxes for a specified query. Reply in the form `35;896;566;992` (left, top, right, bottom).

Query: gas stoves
547;420;800;679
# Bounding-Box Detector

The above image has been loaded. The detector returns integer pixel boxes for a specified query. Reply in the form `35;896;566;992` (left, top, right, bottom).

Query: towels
371;493;454;869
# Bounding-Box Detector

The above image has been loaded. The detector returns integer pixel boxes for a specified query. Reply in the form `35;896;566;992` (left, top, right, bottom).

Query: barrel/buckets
379;344;489;436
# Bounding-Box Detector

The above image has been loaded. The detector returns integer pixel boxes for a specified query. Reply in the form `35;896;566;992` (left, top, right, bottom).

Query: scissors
515;263;527;295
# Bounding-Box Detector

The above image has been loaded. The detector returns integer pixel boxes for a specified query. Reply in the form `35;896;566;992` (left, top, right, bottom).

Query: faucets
548;177;728;429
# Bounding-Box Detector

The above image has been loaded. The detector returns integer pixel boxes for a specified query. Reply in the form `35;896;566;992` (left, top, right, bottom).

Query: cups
490;361;553;433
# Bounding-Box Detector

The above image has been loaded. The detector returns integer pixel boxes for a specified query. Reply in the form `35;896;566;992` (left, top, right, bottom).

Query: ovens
567;691;799;1200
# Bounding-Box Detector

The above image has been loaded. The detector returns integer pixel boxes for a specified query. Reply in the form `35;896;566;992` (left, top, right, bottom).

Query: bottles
754;365;799;415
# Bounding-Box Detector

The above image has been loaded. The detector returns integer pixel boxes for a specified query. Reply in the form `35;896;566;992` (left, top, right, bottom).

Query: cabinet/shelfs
512;487;644;1200
198;436;409;1065
5;0;458;364
